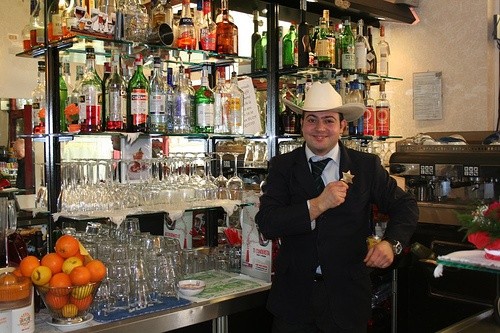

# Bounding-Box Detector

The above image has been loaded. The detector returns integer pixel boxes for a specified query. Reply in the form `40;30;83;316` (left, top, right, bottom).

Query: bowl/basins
177;280;206;296
33;282;101;332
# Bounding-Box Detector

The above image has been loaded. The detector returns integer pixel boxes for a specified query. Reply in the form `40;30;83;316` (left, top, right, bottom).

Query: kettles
411;179;434;202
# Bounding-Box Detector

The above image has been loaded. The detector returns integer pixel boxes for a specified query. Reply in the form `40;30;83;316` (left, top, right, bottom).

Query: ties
309;158;332;198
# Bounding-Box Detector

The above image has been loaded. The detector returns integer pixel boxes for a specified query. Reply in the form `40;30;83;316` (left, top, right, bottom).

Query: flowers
459;203;500;250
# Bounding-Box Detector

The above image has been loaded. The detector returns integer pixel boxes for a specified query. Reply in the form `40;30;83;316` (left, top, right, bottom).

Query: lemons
62;304;78;317
30;266;51;285
62;257;82;274
72;283;93;298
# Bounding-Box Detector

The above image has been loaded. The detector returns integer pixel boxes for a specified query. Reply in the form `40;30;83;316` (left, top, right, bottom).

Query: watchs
381;237;403;256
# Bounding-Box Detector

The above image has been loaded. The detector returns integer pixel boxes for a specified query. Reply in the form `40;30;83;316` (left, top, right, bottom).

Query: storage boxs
239;210;281;282
162;211;208;250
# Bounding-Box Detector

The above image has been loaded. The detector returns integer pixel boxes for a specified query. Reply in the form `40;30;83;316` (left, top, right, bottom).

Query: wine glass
35;152;244;216
281;142;303;154
245;142;269;168
341;139;394;166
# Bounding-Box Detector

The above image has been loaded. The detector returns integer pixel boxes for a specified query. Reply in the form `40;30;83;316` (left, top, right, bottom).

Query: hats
282;82;365;122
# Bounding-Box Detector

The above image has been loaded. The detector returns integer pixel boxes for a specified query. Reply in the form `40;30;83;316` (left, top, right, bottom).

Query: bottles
298;9;310;69
354;19;377;75
281;92;295;134
1;271;34;333
377;27;389;75
346;83;363;139
254;32;268;71
295;83;303;135
283;20;298;70
363;83;375;139
310;10;335;68
375;82;391;136
251;11;263;73
22;0;238;56
32;55;245;134
335;17;357;73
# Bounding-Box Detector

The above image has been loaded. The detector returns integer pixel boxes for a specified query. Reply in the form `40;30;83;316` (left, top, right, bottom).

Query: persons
255;81;419;333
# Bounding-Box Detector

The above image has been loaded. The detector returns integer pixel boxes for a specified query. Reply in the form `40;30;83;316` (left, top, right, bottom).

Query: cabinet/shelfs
16;33;404;223
391;131;500;333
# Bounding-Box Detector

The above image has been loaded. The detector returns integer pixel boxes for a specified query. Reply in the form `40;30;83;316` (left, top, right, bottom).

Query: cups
63;218;241;317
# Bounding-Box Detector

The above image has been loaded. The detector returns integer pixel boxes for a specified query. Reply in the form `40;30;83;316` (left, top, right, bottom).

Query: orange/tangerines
14;234;106;317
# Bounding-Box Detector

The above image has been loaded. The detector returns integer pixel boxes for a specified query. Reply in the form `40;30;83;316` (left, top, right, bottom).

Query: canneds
0;273;35;333
